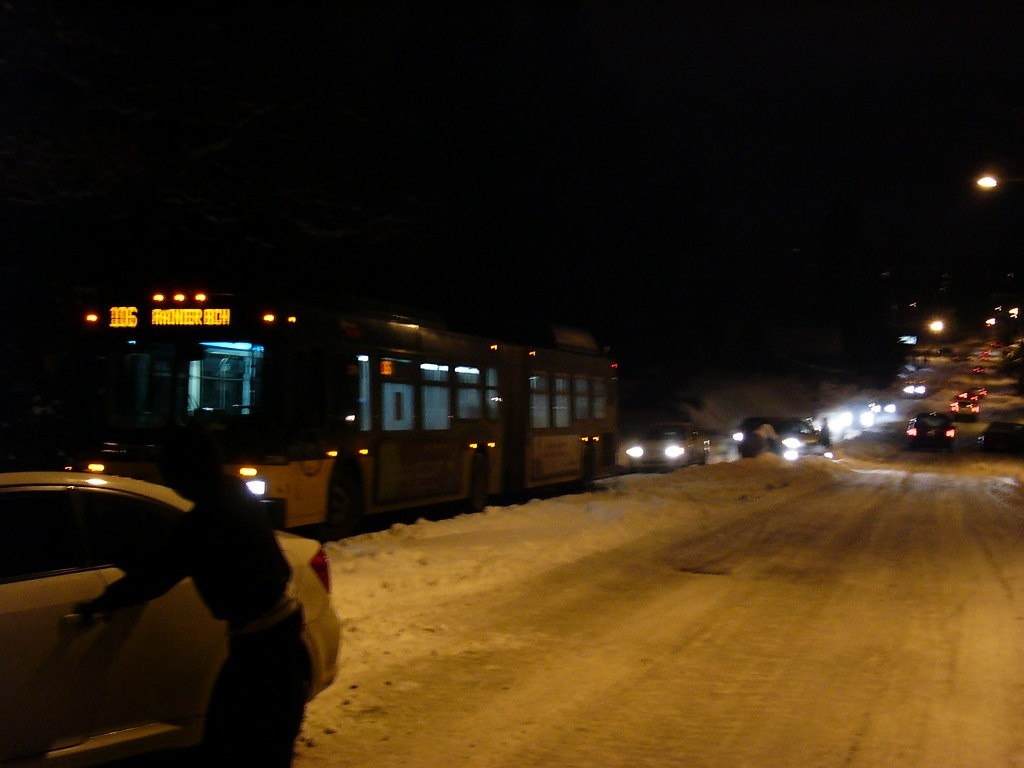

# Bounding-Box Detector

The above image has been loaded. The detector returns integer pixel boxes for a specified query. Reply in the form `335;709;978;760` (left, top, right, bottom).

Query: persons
752;421;778;453
71;428;310;768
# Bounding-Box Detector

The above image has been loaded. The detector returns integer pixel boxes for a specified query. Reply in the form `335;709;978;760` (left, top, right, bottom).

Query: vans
733;415;827;455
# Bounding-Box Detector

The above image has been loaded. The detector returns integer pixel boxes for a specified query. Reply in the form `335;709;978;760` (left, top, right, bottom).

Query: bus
59;285;622;540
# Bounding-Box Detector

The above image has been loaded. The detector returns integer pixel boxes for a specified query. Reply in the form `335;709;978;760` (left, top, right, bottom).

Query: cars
837;338;1024;456
1;469;342;767
626;419;709;472
